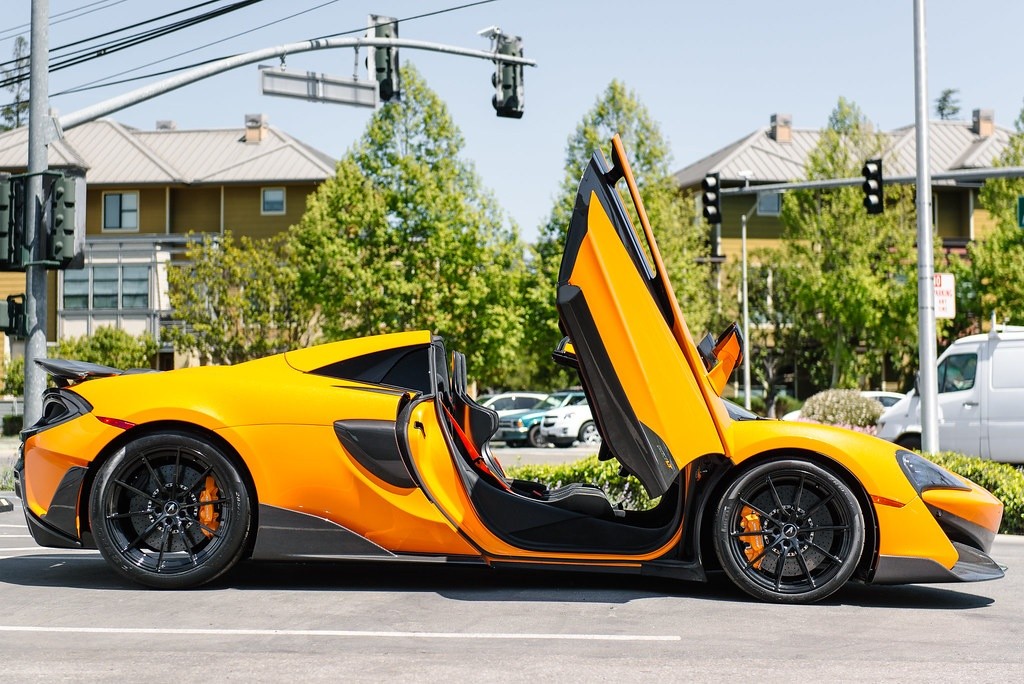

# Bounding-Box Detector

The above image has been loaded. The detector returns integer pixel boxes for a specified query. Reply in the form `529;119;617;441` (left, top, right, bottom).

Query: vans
873;325;1024;473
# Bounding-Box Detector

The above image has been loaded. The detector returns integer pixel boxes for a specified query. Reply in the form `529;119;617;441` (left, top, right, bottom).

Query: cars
539;391;605;451
780;390;907;434
478;389;563;440
495;386;588;449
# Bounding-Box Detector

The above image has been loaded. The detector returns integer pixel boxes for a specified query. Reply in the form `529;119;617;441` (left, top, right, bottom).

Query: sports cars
18;134;1006;623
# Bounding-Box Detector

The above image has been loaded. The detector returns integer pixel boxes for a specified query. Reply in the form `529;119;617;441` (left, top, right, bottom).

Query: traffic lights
861;157;885;215
701;172;723;226
491;34;526;118
